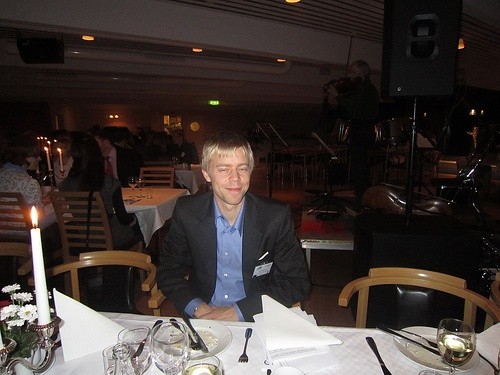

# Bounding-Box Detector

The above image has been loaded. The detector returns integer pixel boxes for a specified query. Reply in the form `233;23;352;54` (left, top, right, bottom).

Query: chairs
47;191;146;289
28;251;166;317
0;192;41;276
339;266;500;329
139;161;176;188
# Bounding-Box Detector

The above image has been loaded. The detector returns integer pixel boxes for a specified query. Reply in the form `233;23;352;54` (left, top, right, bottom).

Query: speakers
16;37;65;65
381;0;464;100
354;209;479;332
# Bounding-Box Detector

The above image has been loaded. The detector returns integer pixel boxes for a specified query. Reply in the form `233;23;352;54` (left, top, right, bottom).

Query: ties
104;156;112;176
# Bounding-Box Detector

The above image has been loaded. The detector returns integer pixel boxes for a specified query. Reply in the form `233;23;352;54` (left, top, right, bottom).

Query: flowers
0;282;56;330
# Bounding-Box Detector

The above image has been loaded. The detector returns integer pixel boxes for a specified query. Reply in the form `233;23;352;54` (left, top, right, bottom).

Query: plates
169;319;232;362
393;326;480;373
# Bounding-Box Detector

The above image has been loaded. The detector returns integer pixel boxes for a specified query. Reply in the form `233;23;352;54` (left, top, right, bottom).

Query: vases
1;320;39;359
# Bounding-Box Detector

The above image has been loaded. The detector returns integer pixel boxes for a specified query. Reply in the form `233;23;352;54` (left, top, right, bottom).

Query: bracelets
194;308;198;312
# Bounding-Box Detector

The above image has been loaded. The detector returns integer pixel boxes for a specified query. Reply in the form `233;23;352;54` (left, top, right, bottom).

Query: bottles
112;342;137;375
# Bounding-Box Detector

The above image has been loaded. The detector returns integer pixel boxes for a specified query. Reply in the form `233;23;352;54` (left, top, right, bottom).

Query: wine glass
128;176;137;199
135;177;145;198
436;318;477;375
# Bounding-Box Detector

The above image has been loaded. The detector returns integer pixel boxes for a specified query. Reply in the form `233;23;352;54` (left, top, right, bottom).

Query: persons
94;131;135;187
157;133;310;322
60;135;144;252
102;127;199;188
0;129;74;233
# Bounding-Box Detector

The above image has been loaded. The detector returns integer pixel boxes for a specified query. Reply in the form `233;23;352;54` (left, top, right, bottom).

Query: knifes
182;315;208;353
366;337;392;375
133;319;163;358
376;325;441;356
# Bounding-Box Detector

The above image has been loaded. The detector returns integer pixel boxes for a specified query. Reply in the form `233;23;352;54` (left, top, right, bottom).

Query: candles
58;148;64;171
37;136;53;171
30;205;50;325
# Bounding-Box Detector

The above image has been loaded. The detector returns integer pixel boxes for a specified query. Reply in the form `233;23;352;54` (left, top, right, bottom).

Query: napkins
253;294;340;364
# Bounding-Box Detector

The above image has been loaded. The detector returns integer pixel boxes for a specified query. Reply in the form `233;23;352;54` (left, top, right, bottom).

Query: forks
170;317;200;350
237;328;252;362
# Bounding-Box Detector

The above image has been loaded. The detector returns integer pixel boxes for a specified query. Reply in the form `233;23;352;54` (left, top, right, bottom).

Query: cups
150;321;190;375
101;343;140;375
146;187;153;199
117;325;152;375
181;352;222;375
271;365;305;375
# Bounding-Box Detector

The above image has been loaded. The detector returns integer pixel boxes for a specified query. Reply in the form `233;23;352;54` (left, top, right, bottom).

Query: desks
0;312;500;375
175;169;198;195
191;163;210;193
121;187;192;248
298;206;357;272
